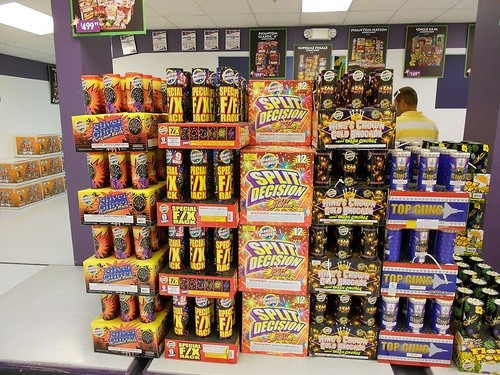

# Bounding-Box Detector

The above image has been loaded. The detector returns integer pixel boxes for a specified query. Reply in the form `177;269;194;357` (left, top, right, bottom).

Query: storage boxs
240;146;313;226
250;79;314;148
238;224;309;296
240;292;310;356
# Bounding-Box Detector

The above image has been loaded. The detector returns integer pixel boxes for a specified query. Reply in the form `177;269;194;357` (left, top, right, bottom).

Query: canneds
420;139;500;340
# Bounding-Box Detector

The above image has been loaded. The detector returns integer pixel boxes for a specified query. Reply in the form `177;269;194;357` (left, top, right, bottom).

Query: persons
394;86;440;142
